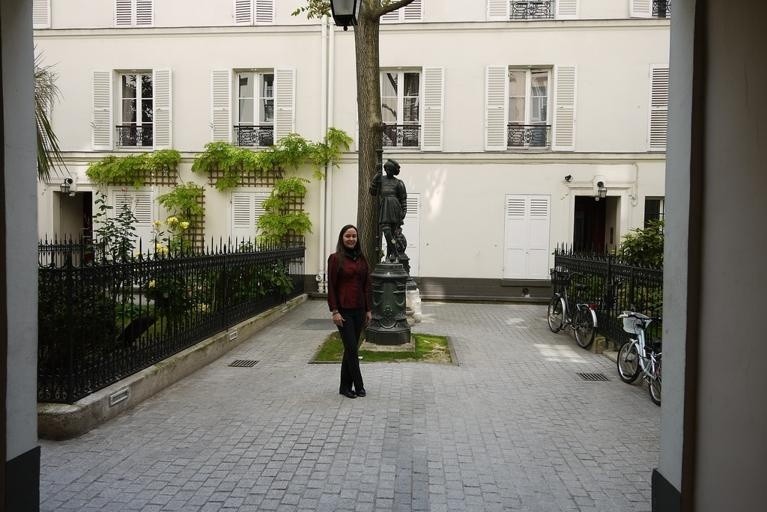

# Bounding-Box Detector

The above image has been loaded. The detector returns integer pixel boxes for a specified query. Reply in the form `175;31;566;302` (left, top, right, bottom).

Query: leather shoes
339;385;366;399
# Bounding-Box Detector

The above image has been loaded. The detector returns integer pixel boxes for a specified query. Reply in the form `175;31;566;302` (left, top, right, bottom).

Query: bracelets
333;312;339;316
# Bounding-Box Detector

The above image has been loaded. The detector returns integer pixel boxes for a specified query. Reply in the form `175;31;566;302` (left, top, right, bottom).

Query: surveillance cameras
565;175;571;182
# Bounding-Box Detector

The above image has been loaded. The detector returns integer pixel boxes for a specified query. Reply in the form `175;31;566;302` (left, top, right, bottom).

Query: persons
370;159;408;264
328;225;372;398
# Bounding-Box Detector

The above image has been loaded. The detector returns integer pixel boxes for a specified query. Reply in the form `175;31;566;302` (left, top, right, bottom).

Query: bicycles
548;269;598;348
616;310;662;406
